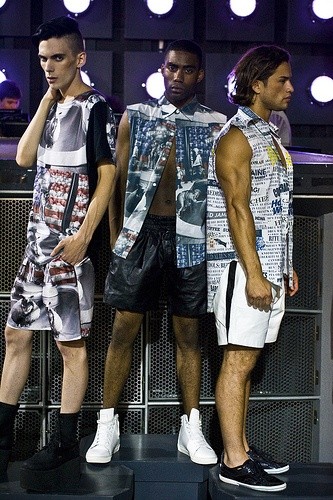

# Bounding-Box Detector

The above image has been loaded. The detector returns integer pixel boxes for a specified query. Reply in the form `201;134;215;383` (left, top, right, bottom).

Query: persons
0;16;118;489
0;79;21;109
204;46;297;489
85;40;226;464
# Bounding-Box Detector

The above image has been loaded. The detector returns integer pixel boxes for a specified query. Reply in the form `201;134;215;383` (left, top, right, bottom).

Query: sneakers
177;408;218;465
220;446;289;475
218;458;286;492
85;408;120;463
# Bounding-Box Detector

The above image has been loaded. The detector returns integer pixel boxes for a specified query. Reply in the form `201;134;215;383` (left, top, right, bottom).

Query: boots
22;410;80;471
0;402;20;454
